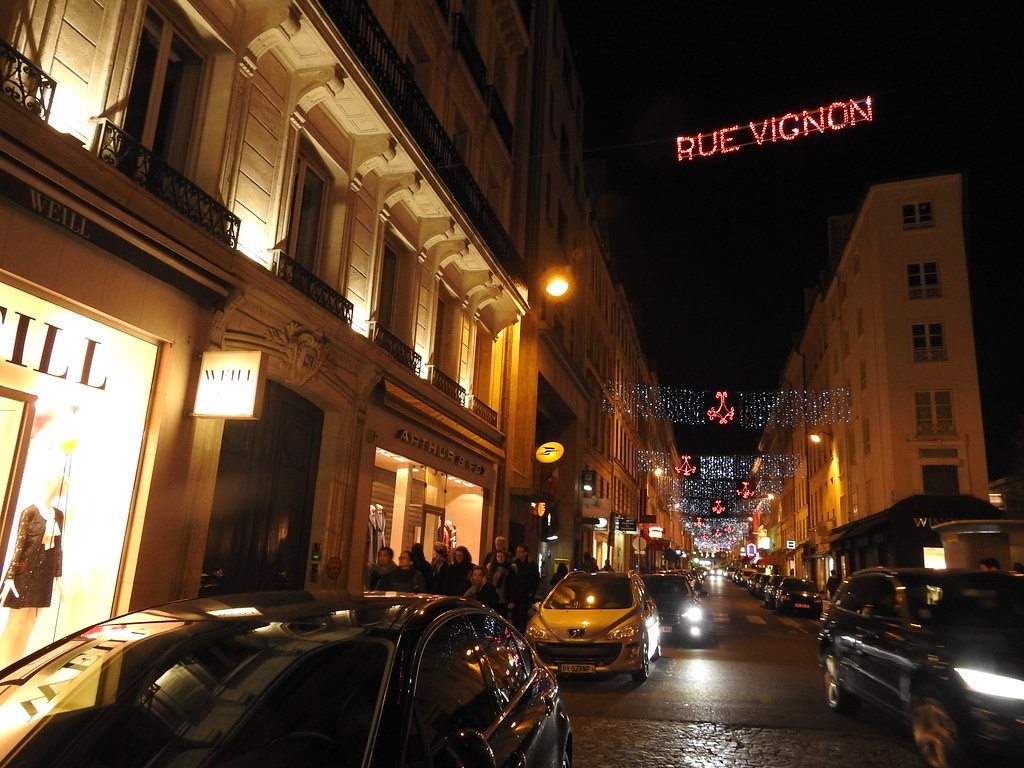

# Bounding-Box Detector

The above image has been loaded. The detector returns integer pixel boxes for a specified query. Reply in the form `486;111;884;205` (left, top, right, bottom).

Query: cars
819;566;1024;768
640;565;709;644
775;576;824;619
0;582;578;768
710;565;763;593
524;570;662;683
754;574;772;599
764;574;784;609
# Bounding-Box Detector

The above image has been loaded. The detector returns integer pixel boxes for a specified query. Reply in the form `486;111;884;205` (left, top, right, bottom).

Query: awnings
794;518;886;558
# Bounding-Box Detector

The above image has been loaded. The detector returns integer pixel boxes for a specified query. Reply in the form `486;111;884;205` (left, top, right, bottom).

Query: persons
825;569;840;600
366;537;614;636
437;520;456;565
0;457;70;671
979;558;1000;571
366;504;387;563
1012;562;1024;574
